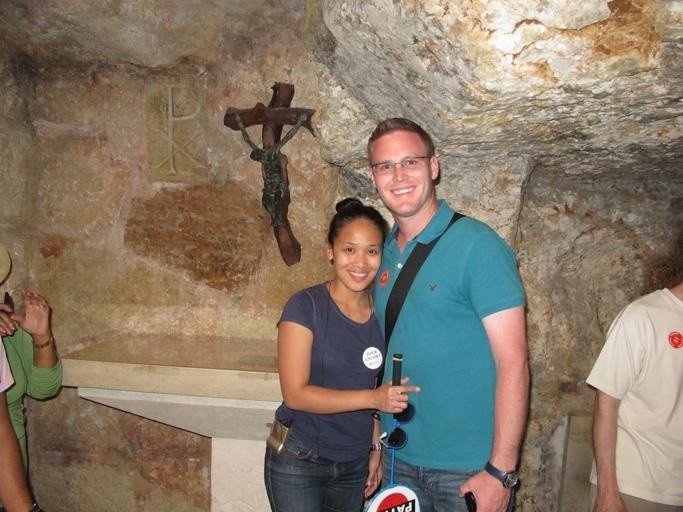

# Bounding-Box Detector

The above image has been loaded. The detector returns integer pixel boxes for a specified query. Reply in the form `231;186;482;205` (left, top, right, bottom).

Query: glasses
370;156;432;176
378;403;415;449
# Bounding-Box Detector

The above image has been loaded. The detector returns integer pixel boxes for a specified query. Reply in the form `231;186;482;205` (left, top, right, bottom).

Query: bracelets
30;335;53;351
368;443;382;452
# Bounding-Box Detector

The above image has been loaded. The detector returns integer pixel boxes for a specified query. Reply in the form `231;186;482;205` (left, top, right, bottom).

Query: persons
0;243;64;485
262;195;422;512
0;339;53;511
363;115;532;512
581;273;682;511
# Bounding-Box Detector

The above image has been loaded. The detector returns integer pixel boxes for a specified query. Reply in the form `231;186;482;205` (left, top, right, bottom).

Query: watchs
482;461;520;490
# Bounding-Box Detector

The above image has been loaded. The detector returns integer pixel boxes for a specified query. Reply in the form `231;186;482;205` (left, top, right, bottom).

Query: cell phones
463;491;477;512
